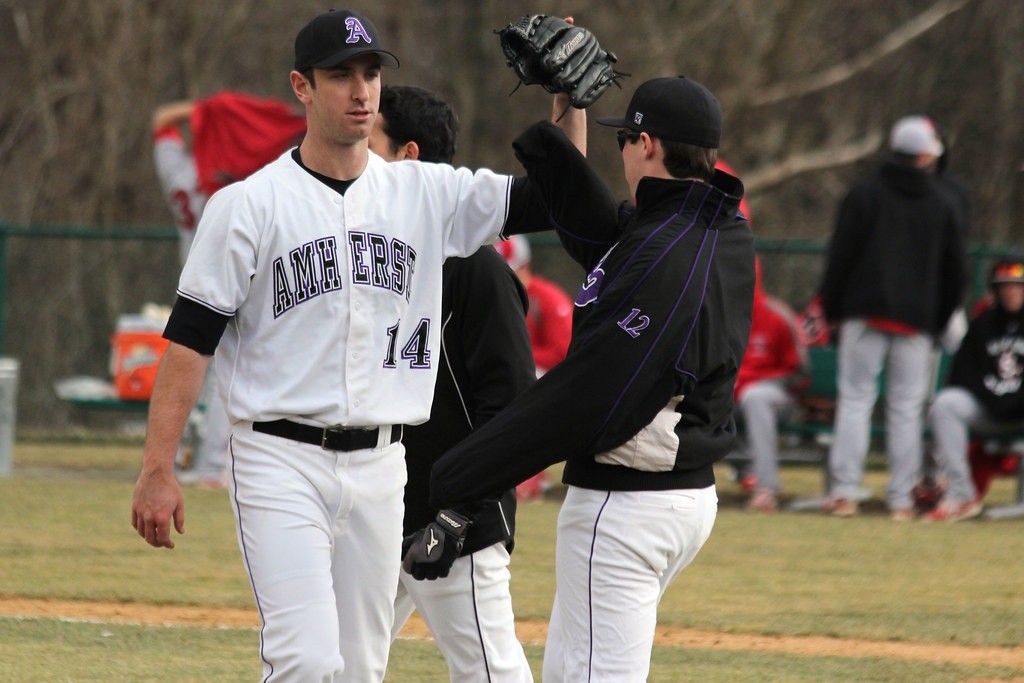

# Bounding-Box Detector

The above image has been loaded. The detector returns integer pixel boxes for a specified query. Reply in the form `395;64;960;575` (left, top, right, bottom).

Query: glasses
616;131;640;151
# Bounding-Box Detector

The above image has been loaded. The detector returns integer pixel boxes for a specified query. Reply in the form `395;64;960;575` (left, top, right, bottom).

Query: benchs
71;337;957;475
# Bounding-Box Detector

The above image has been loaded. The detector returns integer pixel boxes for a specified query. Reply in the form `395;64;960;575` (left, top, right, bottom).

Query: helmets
490;234;532;272
987;260;1024;283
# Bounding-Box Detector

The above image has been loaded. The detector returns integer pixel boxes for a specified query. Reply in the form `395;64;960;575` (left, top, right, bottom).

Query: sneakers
920;496;983;524
739;475;756;490
889;508;917;523
513;476;552;502
822;498;859;517
743;488;779;512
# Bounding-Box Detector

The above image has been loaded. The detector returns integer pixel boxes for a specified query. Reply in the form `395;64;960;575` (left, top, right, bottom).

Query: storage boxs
111;304;180;400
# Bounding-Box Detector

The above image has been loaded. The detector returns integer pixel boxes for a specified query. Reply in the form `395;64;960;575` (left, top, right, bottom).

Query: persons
150;91;308;468
401;74;756;683
481;231;575;500
734;299;807;512
818;114;964;518
132;11;588;683
919;259;1024;521
366;82;536;682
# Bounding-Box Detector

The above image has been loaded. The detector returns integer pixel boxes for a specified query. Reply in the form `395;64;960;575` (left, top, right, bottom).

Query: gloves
405;509;476;581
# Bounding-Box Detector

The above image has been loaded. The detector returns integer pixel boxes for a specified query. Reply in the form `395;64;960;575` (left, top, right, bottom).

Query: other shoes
199;481;225;491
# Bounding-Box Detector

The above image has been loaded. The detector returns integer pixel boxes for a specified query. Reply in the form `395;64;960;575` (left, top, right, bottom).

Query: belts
250;419;400;452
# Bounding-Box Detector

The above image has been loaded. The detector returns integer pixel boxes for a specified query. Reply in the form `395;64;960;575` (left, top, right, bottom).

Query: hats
595;75;722;148
293;8;399;79
889;116;945;157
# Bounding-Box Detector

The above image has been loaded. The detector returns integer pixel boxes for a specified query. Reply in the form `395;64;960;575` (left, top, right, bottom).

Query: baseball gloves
500;12;616;111
